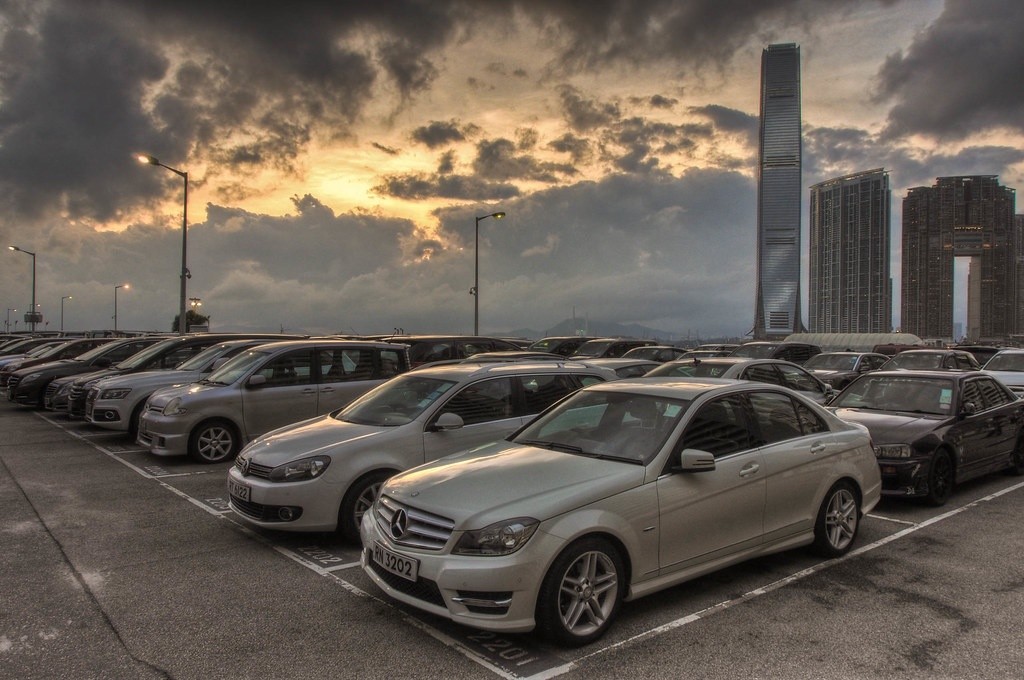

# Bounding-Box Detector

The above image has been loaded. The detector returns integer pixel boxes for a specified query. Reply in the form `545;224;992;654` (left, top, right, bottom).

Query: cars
226;362;621;535
360;372;884;651
1;327;1024;465
830;370;1024;506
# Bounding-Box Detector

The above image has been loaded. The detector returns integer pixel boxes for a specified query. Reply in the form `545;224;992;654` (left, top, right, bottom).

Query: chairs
627;398;656;430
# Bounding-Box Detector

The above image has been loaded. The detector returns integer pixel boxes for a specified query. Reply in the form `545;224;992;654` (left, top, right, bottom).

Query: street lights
61;296;72;330
8;246;36;331
7;308;17;333
115;285;130;331
189;297;202;312
475;210;507;336
29;303;40;331
135;153;188;337
